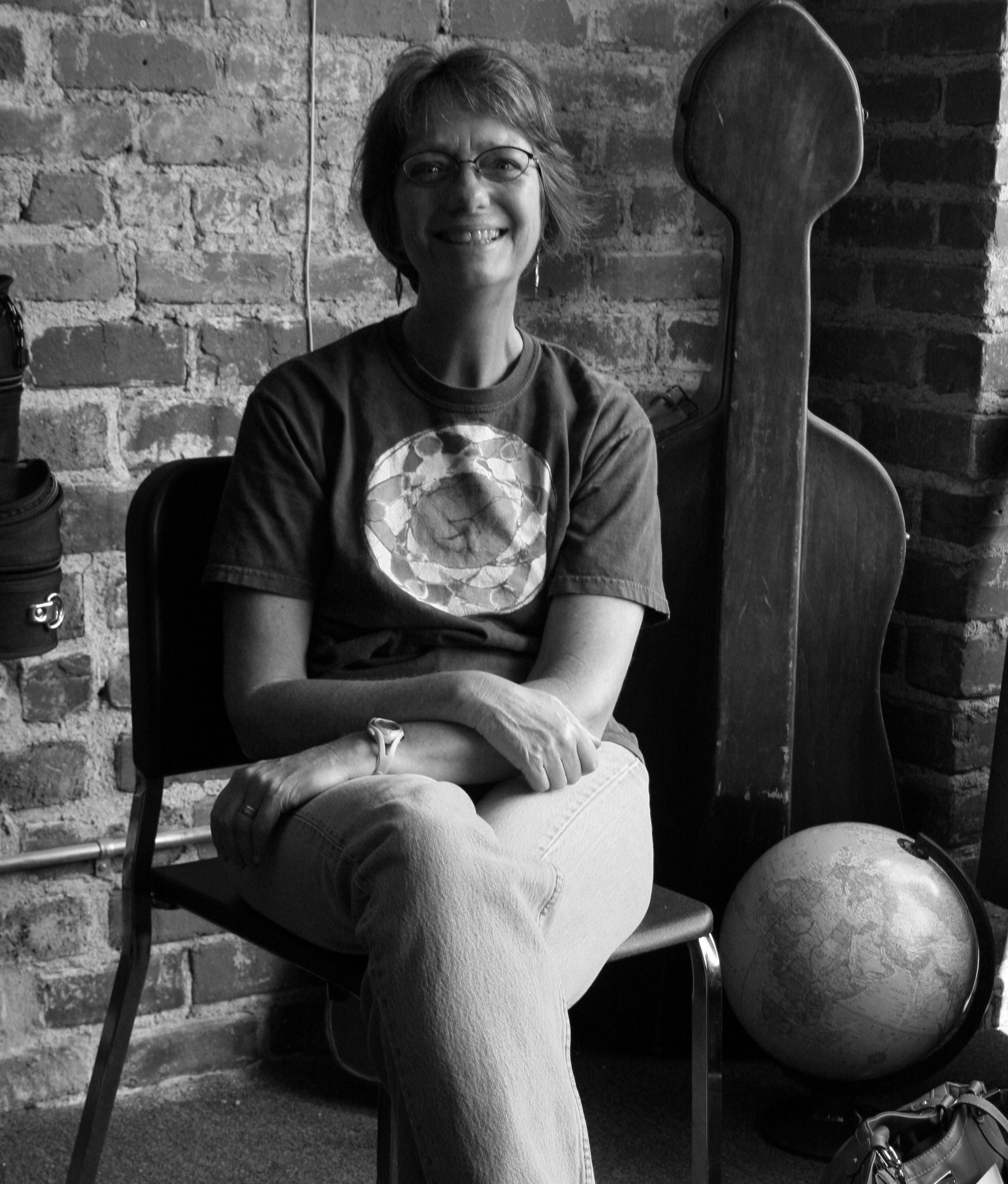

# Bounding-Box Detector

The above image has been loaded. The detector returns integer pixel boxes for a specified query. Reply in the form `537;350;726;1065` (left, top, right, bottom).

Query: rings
240;803;257;818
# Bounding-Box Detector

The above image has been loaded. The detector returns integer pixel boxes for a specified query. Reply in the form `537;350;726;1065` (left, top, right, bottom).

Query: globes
719;822;997;1162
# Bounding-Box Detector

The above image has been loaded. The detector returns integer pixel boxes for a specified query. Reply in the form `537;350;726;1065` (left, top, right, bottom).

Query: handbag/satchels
827;1080;1008;1184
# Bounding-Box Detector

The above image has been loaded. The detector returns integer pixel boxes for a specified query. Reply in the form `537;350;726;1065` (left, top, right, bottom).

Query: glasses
397;145;540;190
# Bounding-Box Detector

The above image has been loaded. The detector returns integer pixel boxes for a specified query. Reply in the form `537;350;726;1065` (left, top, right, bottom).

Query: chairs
64;456;725;1184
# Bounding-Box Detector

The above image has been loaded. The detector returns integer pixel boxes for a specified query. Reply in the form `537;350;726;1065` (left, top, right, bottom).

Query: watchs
367;712;404;773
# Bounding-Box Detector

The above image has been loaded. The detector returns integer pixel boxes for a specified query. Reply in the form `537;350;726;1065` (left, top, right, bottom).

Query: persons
211;37;674;1184
